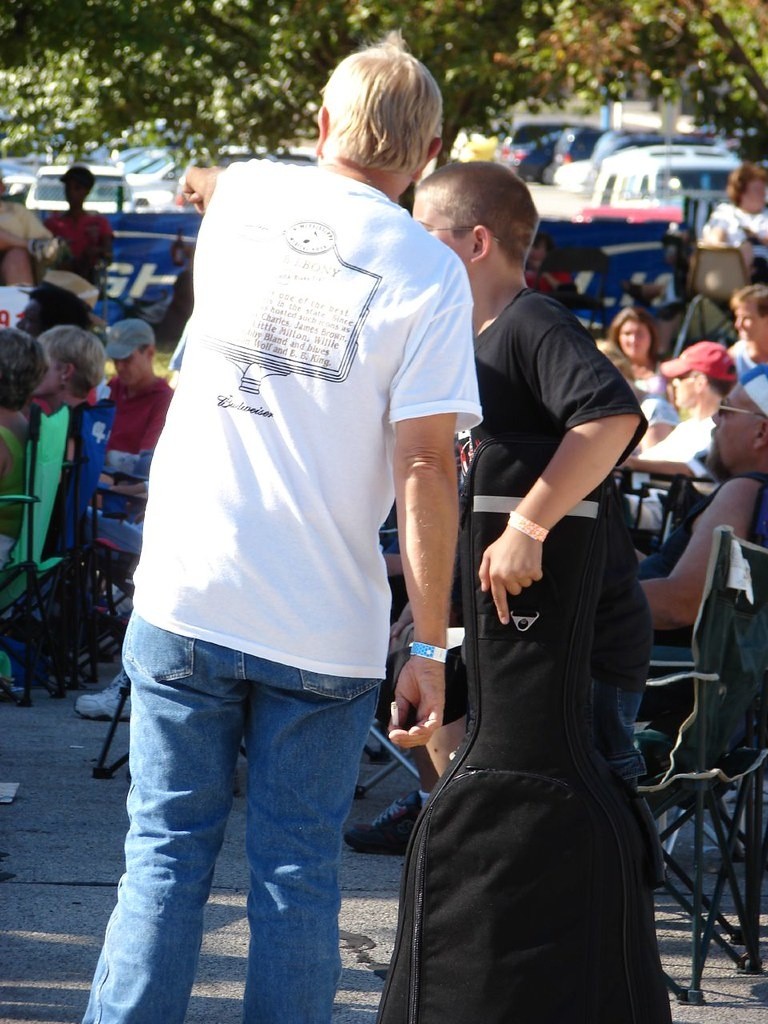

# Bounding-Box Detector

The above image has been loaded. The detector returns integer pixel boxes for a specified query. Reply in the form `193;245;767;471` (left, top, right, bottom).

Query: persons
411;161;654;793
609;307;677;409
0;177;54;285
30;325;104;462
725;284;767;374
43;166;113;278
0;325;48;569
595;341;680;453
702;163;768;284
618;341;737;561
85;319;176;554
75;501;402;722
345;363;768;856
82;45;483;1024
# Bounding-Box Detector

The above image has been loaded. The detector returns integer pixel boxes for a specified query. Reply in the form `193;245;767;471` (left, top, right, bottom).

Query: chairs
639;523;768;1007
671;241;752;359
0;397;155;706
535;247;609;333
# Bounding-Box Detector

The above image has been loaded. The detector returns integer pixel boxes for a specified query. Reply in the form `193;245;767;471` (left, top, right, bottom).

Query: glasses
718;397;768;422
670;374;699;387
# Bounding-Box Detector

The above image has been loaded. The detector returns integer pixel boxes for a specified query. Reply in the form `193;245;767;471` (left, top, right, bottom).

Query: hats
104;318;155;360
658;343;737;382
60;167;94;188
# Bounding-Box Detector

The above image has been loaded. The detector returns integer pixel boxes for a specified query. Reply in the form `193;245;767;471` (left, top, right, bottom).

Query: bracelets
507;511;548;542
410;642;446;664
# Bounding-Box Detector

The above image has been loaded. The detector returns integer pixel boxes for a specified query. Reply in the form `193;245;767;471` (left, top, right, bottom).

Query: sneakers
345;789;424;853
74;668;131;722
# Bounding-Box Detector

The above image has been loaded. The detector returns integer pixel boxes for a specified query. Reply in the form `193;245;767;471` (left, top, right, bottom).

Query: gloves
30;236;77;269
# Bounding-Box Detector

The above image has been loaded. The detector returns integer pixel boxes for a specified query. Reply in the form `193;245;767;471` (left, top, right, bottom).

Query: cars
0;117;744;224
